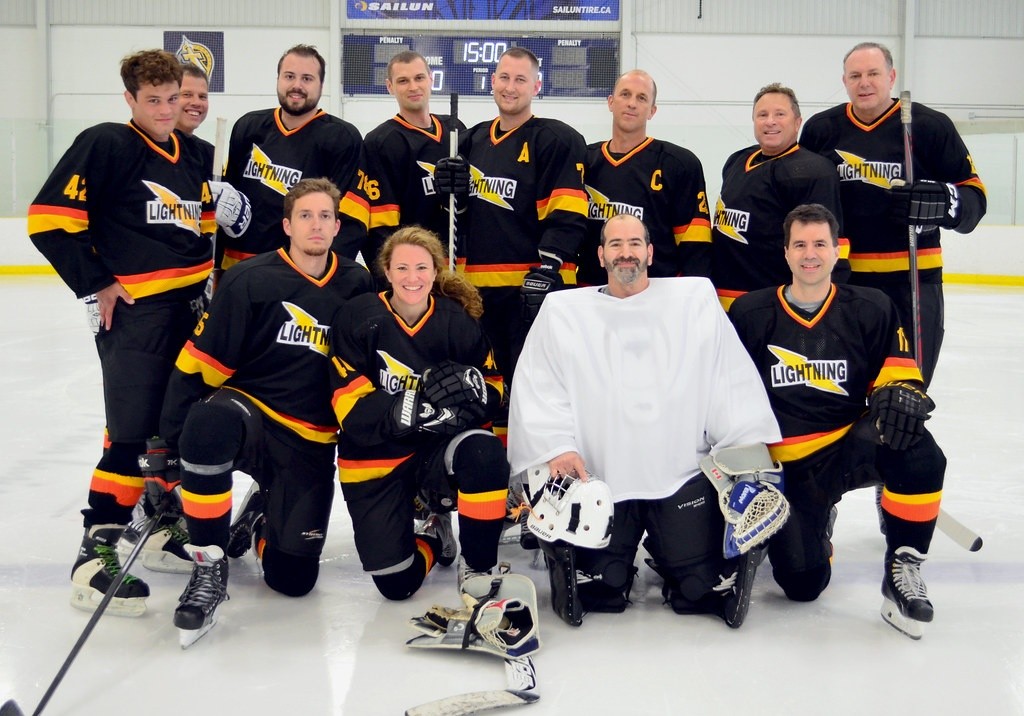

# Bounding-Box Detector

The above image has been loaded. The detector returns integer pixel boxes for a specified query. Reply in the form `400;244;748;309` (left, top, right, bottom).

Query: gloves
519;267;565;324
871;382;936;452
393;390;486;437
208;180;253;237
433;155;471;214
137;438;183;526
886;178;963;233
424;359;499;419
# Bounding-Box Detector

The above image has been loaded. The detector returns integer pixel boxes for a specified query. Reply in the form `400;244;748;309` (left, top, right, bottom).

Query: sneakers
173;544;230;649
413;490;431;521
70;528;151;618
115;502;152;558
508;503;543;567
881;545;935;640
500;487;527;543
828;506;841;564
457;553;493;600
417;512;456;567
141;519;196;575
226;482;268;560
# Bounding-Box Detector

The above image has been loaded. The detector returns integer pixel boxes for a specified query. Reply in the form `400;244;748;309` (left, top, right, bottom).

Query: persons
214;43;363;286
324;226;509;600
584;70;712;286
796;43;987;398
710;83;853;317
171;65;211;132
503;211;791;627
27;50;215;616
353;48;467;291
442;48;591;565
138;176;373;651
724;202;947;642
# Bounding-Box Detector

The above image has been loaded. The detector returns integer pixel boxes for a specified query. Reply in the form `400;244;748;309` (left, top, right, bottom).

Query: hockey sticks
0;515;161;715
211;115;227;203
449;90;459;273
899;89;987;552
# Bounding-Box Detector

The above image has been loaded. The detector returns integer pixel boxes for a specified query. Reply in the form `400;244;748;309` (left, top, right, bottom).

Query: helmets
526;467;614;548
